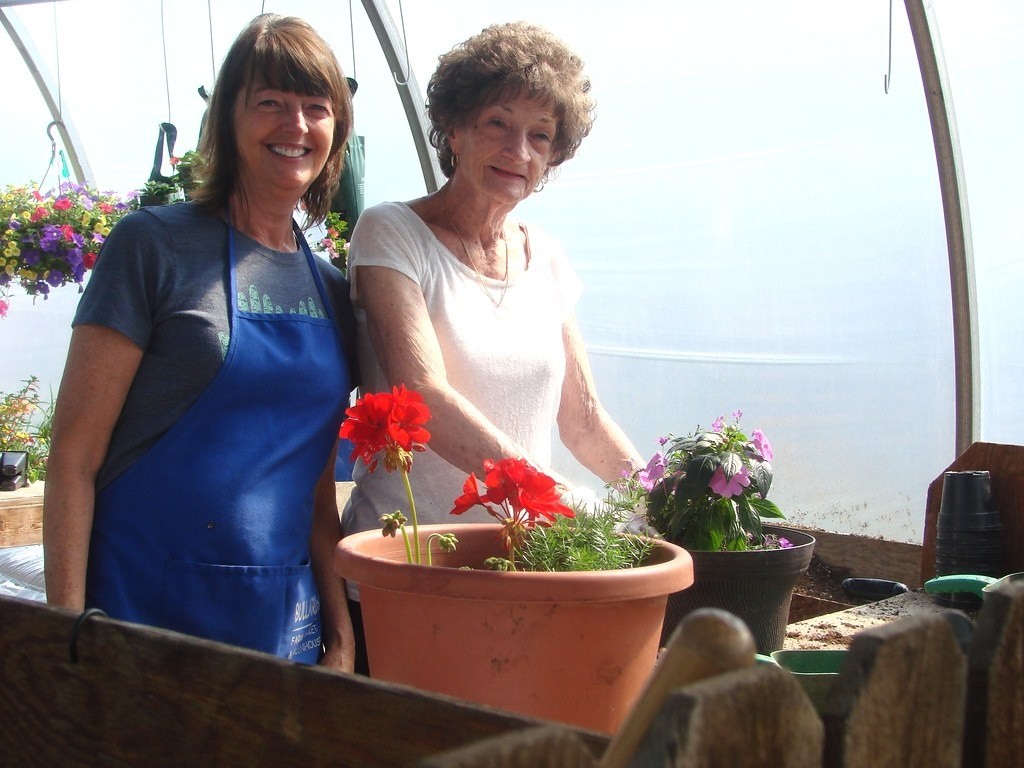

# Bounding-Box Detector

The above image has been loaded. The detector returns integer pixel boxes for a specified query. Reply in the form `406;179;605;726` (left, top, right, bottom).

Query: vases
765;647;851;718
332;523;695;738
0;450;28;489
623;516;815;662
936;470;1003;607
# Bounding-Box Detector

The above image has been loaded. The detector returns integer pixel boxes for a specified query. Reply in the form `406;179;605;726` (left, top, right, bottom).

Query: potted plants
137;180;178;208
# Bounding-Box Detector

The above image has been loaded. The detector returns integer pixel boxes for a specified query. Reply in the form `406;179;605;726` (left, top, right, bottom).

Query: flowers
611;410;795;551
338;382;659;575
1;181;131;312
290;198;351;260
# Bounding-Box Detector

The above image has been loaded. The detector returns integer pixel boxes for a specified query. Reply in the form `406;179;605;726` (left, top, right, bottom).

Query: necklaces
440;186;509;308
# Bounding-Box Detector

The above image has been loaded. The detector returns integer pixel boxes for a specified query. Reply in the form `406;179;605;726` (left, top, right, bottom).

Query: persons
43;12;359;678
339;21;651;681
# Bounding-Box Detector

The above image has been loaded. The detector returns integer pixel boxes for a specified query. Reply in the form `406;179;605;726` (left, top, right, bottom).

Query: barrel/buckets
935;470;1003;605
